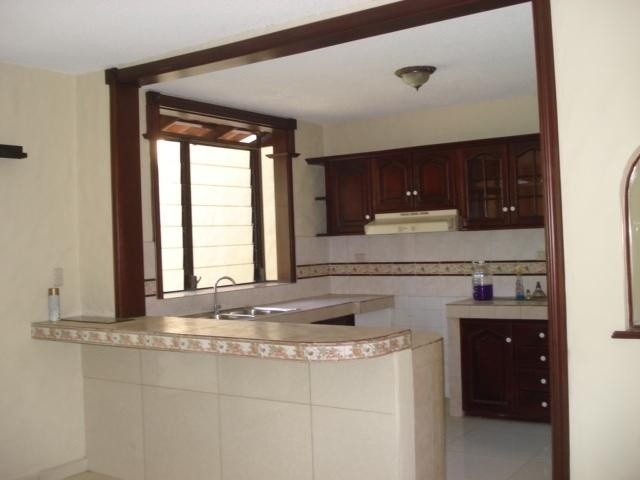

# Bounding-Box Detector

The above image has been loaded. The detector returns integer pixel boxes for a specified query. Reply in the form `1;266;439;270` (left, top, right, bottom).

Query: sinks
492;298;548;307
226;307;301;316
187;314;256;321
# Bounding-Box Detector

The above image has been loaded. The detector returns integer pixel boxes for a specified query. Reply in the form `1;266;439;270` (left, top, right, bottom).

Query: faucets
532;281;546;298
214;276;236;310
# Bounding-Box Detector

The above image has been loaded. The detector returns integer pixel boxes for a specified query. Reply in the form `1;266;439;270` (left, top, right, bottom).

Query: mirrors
609;145;639;341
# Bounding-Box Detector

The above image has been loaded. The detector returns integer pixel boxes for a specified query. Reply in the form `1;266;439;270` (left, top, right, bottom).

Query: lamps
394;66;436;92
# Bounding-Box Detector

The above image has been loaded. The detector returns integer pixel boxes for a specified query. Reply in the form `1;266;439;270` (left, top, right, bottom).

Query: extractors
363;208;458;235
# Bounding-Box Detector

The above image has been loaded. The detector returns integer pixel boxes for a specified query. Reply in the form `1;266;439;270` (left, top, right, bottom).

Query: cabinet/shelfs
305;155;375;238
454;130;545;230
371;147;454;213
310;314;356;327
459;317;550;421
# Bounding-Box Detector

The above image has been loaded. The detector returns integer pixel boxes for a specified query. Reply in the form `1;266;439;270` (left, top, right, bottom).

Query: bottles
515;273;525;300
533;281;545;297
525;289;531;300
